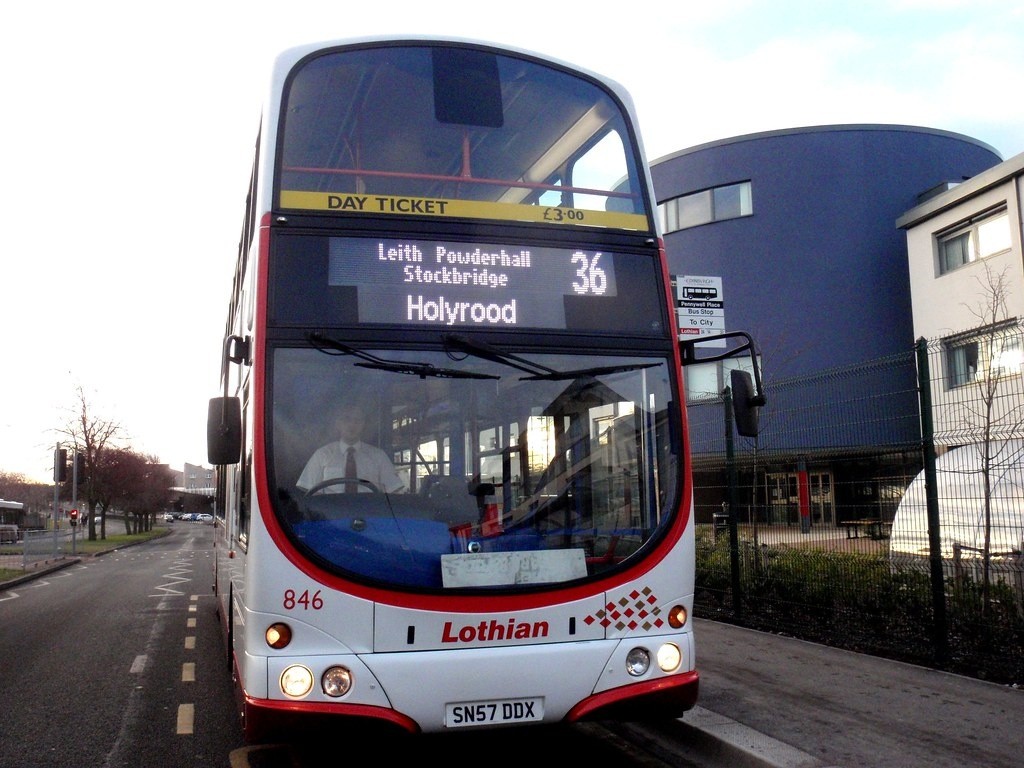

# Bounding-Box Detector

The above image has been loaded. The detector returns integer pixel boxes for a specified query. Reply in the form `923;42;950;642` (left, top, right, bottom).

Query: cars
166;515;173;522
169;511;214;521
95;517;101;523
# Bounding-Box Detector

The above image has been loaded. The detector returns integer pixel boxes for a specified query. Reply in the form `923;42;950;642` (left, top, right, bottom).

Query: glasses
338;415;365;425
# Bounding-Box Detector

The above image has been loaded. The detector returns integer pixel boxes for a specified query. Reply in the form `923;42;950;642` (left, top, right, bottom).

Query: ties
345;446;358;495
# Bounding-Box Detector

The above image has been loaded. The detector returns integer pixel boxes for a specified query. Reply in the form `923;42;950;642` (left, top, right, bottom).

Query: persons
294;398;405;494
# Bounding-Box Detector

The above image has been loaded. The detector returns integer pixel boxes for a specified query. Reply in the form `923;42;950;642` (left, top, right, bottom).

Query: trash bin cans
712;513;732;544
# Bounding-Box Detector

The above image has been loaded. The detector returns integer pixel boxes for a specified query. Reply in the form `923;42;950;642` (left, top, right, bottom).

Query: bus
208;34;767;734
0;499;26;544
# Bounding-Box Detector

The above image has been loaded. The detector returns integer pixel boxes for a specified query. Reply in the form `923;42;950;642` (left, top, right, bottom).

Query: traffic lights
70;510;77;526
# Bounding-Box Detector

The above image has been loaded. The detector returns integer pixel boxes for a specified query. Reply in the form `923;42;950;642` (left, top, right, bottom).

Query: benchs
841;521;892;539
